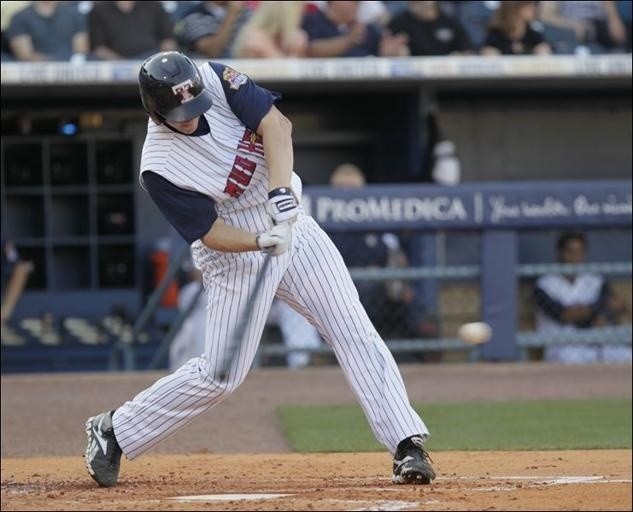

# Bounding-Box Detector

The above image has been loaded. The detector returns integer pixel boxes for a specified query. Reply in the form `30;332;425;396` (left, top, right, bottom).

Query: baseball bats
213;242;273;381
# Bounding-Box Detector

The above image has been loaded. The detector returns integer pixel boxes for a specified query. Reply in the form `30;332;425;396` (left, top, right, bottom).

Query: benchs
435;275;633;362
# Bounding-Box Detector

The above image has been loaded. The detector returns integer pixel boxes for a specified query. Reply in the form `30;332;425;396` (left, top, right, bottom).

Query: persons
2;1;632;59
0;234;35;328
83;51;436;488
531;231;633;362
166;273;319;374
318;159;442;365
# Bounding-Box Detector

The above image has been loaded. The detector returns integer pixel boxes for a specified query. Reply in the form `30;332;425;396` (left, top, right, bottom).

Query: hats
139;49;213;124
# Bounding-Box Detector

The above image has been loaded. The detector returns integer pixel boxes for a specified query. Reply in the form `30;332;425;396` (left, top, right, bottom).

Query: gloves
264;186;299;225
254;225;294;256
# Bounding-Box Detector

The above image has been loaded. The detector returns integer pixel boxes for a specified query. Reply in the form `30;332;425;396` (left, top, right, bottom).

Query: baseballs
460;321;491;346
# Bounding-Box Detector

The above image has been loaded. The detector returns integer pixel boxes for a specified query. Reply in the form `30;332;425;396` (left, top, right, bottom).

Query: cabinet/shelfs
0;124;177;293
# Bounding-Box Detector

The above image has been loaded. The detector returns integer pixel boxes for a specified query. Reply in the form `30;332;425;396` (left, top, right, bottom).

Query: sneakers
83;408;124;490
389;436;437;486
